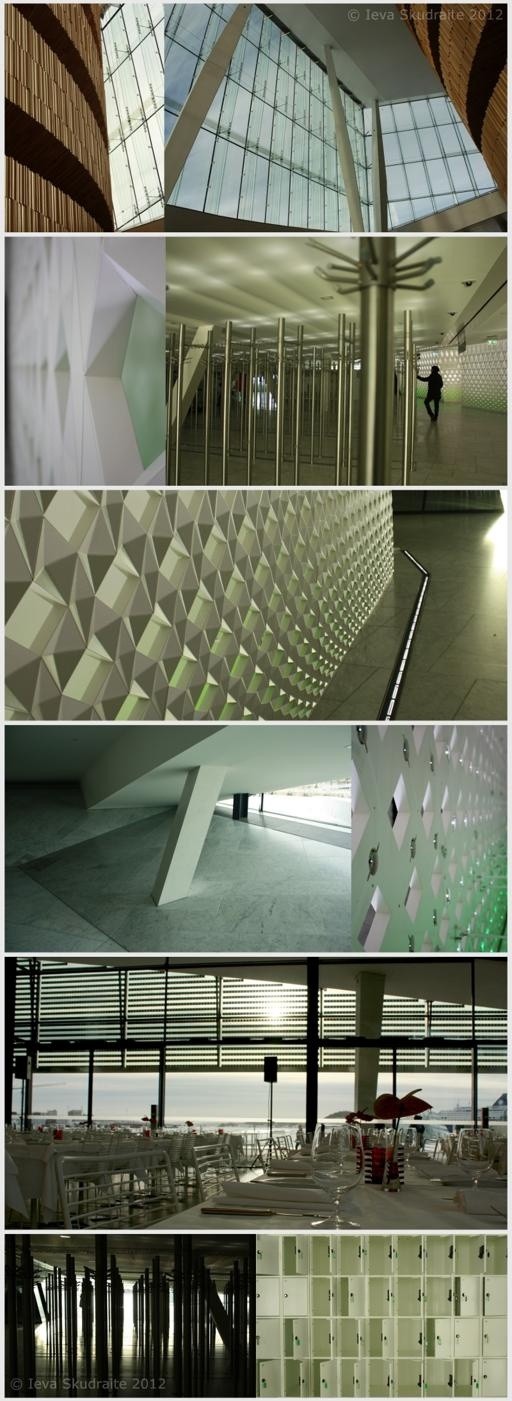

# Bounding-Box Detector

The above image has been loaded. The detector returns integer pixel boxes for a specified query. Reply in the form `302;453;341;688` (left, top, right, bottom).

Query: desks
5;1132;507;1229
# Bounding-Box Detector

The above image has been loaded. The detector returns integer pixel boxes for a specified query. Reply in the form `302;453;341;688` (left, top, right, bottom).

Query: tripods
250;1082;287;1175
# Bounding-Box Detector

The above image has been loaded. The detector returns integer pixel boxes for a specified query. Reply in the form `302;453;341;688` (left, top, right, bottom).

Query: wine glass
307;1122;496;1230
5;1122;17;1143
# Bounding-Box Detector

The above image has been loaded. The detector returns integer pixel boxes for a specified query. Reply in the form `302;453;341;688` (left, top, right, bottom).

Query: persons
411;1116;425;1151
418;366;443;421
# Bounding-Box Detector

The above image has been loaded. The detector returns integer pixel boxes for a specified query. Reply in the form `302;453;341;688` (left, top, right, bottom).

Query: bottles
408;1114;425;1151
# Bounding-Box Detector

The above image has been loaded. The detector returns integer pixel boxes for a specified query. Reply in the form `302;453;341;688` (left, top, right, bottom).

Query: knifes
201;1206;331;1218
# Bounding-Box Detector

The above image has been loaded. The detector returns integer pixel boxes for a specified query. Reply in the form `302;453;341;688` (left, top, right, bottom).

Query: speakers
264;1057;277;1082
15;1056;32;1079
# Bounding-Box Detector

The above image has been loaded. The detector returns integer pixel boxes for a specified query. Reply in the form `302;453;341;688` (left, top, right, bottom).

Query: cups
144;1129;150;1136
55;1123;65;1139
382;1126;403;1193
